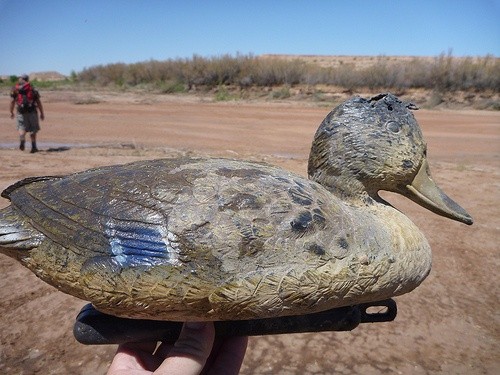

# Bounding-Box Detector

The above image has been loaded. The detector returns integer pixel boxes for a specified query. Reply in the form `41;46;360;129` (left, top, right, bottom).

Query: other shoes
19;140;25;150
31;148;37;152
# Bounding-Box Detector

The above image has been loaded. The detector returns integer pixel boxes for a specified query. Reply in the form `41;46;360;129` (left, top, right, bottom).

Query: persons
10;74;44;153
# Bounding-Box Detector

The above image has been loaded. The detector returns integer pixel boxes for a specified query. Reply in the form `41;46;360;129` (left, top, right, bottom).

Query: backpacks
14;80;34;113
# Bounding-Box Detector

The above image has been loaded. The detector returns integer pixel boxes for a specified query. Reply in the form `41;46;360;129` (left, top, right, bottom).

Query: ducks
1;94;474;323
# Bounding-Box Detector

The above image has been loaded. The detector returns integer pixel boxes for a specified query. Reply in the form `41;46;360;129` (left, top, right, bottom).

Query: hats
22;74;29;79
